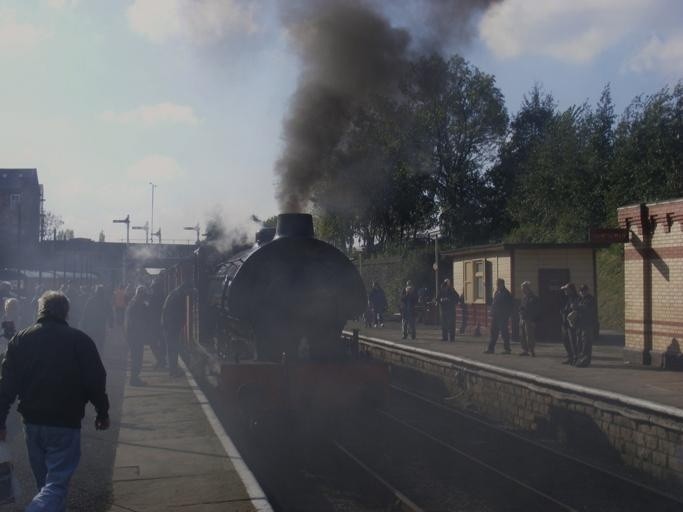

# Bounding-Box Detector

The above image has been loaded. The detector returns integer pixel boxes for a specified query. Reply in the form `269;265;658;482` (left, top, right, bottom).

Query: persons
435;278;460;342
570;284;596;368
558;282;581;366
482;278;514;355
516;280;539;357
399;280;419;339
366;281;389;327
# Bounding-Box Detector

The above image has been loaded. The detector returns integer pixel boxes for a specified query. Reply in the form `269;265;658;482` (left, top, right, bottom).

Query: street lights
147;179;156;258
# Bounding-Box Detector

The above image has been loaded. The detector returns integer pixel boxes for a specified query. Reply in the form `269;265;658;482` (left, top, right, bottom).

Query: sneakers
560;352;592;369
482;349;536;359
374;323;456;344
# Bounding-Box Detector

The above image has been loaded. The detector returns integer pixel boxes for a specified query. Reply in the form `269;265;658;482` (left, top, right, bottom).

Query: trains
160;213;395;413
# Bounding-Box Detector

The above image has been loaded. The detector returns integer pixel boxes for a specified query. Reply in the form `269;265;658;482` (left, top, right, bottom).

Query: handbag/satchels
567;310;579;328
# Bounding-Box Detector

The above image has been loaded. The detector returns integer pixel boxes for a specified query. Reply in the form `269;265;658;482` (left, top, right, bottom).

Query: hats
561;283;575;290
